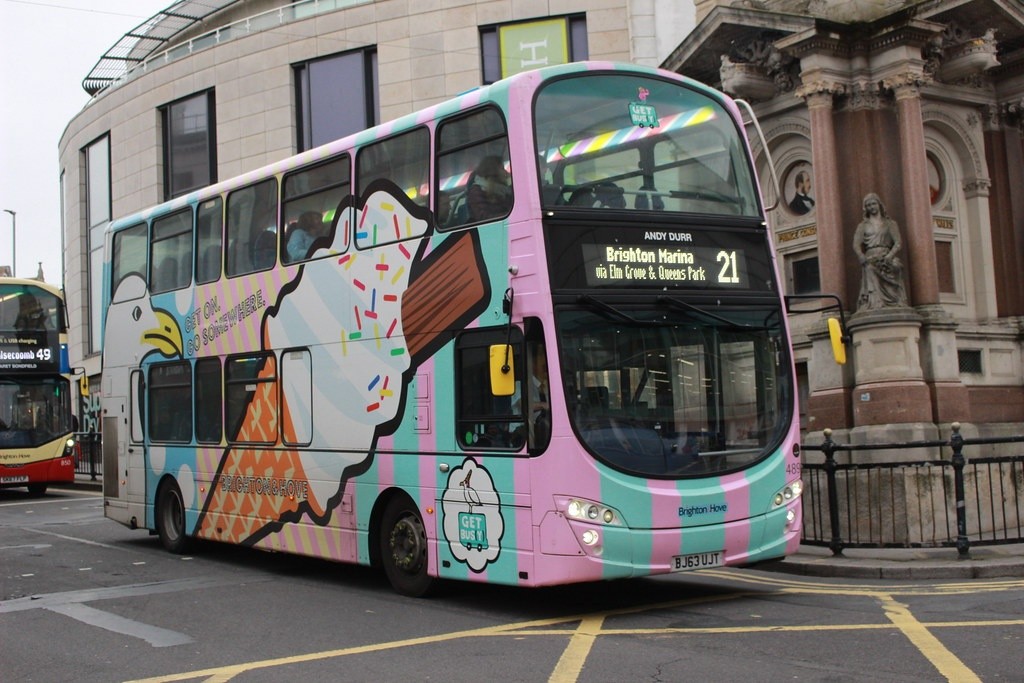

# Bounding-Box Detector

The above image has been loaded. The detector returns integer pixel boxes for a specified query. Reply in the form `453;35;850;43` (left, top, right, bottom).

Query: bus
98;63;857;611
0;277;77;497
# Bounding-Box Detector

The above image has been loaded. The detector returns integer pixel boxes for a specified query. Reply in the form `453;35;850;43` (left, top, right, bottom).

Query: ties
540;387;548;415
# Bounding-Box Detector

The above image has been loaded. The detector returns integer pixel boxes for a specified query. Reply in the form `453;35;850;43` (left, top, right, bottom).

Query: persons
539;154;627;209
635;186;665;209
466;156;513;221
853;193;906;311
72;414;79;468
509;344;548;433
287;212;323;261
427;191;451;222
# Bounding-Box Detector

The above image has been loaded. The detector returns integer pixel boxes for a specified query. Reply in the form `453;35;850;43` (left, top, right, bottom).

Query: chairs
228;238;249;275
155;257;179;292
177;251;193;286
199;245;223;282
458;170;479;224
140;264;155;289
254;230;277;268
636;186;665;209
283;222;299;262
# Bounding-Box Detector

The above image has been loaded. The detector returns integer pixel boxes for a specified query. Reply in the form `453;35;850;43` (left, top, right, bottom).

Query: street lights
4;208;16;276
